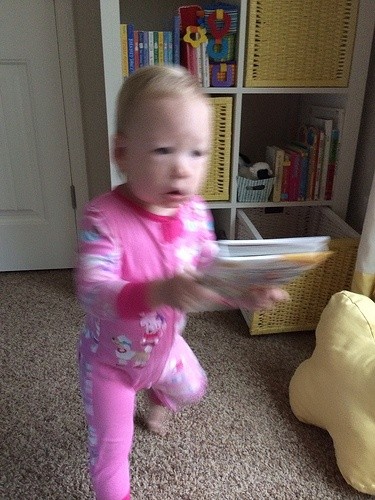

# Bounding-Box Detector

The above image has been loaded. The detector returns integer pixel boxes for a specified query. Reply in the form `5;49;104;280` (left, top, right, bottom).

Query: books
268;105;344;203
120;5;240;88
185;234;332;318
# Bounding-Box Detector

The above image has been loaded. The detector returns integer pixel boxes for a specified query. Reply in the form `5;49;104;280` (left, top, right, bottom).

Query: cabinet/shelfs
73;0;375;336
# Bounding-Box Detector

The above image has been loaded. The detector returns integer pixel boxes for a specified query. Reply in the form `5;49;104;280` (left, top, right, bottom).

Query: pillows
287;291;375;496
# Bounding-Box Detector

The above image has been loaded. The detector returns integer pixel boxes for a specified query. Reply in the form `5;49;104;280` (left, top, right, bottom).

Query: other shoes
144;406;166;432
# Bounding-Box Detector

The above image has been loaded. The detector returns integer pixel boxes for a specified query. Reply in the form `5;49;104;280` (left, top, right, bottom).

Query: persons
74;63;290;499
